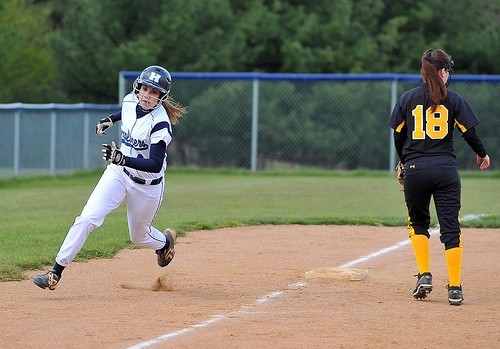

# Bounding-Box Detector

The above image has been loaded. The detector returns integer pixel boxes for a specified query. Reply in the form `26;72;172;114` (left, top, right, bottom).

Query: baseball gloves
395;161;405;187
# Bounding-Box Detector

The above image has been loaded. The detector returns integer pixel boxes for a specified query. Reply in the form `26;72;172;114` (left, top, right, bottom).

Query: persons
388;49;490;305
33;66;183;291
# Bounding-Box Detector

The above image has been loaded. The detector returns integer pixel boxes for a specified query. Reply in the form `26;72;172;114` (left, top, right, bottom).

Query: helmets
134;66;171;109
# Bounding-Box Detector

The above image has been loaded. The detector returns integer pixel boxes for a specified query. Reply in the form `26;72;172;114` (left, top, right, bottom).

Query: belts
123;167;163;185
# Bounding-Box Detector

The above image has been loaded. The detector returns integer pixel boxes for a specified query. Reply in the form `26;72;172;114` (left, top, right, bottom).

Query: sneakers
446;284;463;305
413;272;433;299
155;228;176;267
34;269;60;290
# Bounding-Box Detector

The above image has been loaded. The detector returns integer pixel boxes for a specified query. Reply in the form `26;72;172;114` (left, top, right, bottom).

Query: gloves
101;141;126;166
96;114;117;136
